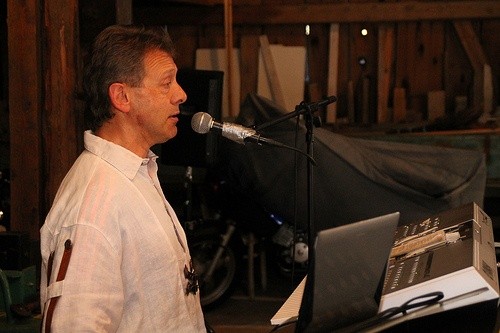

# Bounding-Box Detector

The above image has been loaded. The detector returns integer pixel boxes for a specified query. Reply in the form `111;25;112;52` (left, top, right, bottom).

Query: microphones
191;112;284;149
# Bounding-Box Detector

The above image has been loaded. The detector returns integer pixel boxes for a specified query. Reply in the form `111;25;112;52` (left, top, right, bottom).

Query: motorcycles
171;145;310;311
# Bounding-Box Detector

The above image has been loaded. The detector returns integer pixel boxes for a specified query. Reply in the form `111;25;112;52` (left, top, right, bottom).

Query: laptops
274;211;400;333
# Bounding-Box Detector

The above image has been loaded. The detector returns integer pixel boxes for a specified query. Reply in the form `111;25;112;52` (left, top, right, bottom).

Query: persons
39;26;208;333
